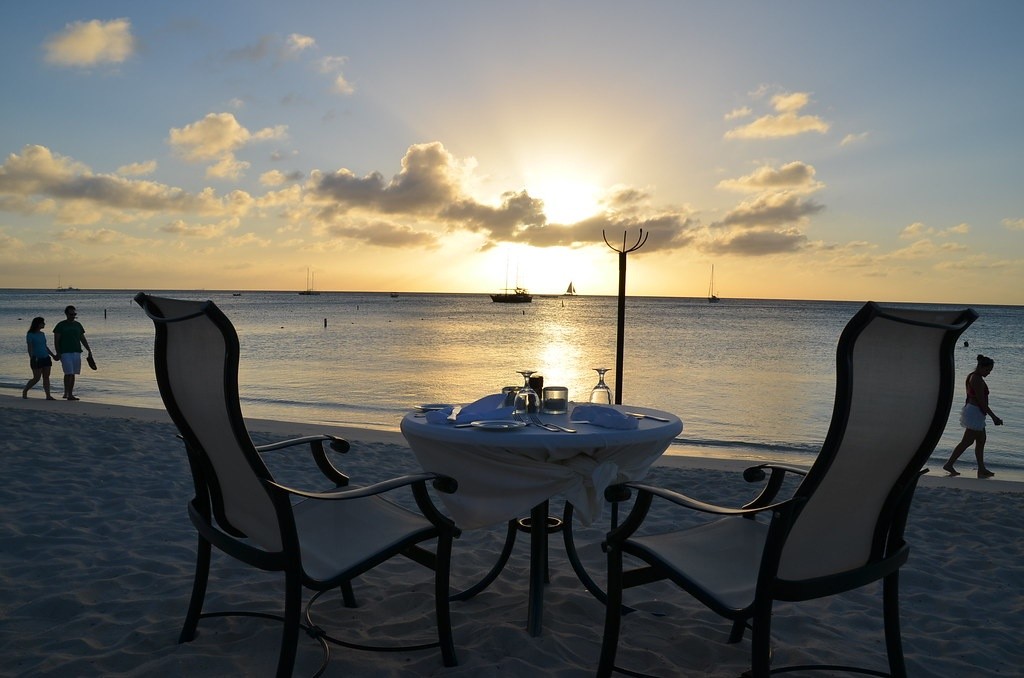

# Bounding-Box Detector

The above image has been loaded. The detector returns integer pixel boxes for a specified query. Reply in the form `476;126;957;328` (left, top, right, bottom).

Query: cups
543;386;568;415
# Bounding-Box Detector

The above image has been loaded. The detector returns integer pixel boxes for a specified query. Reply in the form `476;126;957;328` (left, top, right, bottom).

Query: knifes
624;411;670;423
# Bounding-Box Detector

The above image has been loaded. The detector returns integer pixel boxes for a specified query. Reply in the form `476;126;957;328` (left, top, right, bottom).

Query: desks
401;401;682;639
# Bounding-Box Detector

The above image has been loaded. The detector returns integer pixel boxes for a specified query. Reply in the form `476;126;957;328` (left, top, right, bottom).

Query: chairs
598;300;981;678
133;291;466;678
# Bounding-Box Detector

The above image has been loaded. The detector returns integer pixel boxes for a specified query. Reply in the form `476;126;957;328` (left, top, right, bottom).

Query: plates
413;404;456;411
470;420;527;431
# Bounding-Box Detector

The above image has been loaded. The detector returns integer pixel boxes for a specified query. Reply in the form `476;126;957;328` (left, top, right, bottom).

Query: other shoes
87;356;97;370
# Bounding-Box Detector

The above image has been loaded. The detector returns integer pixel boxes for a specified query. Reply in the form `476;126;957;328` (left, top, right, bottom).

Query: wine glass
589;368;615;405
512;370;540;417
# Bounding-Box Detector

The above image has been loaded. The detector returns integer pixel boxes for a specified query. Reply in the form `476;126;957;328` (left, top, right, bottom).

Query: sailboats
563;281;577;296
299;268;319;295
708;264;720;303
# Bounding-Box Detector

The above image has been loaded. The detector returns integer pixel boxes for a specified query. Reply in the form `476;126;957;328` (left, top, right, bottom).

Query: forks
512;414;560;432
530;415;575;434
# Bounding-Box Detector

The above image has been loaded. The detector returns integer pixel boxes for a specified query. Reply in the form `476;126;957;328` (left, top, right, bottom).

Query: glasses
68;312;77;316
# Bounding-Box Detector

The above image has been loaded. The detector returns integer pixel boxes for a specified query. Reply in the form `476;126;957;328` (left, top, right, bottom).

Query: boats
489;288;533;304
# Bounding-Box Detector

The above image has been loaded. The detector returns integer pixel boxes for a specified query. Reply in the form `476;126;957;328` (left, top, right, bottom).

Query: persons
23;316;59;400
52;305;98;401
943;354;1003;476
